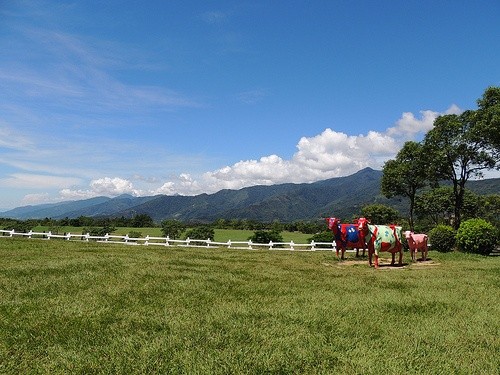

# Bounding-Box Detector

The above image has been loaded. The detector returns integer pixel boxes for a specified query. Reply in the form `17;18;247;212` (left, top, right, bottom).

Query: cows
325;217;368;259
355;218;403;269
402;231;428;261
